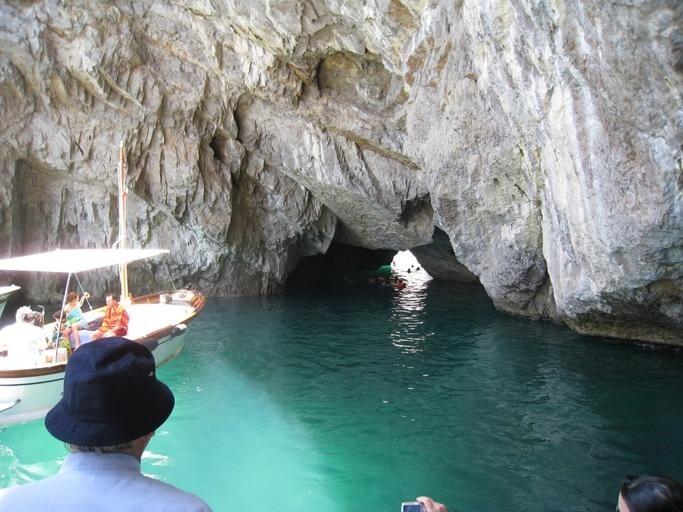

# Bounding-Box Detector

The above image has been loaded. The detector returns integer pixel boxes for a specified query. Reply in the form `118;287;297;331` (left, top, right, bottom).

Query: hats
45;337;174;447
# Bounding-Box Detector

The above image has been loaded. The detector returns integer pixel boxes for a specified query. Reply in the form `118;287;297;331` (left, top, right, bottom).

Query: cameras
401;502;427;512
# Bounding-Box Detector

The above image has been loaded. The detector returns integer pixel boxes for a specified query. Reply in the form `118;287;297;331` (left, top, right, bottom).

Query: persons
62;290;91;352
0;306;49;370
32;311;50;348
415;472;683;512
387;261;420;288
44;309;72;362
89;293;129;341
0;336;212;512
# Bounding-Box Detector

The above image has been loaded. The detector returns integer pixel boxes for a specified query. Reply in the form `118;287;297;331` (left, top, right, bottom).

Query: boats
369;264;406;301
0;147;208;420
